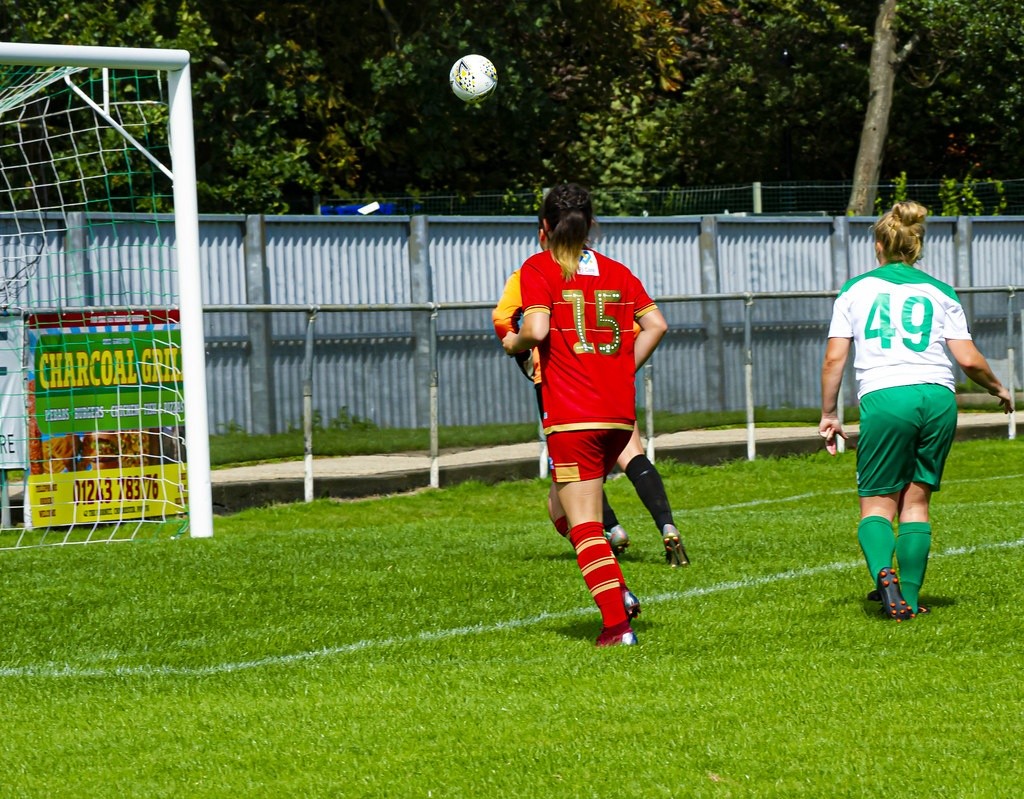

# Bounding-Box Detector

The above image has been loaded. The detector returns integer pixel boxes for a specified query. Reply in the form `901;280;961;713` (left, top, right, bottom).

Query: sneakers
876;567;915;623
594;627;640;648
661;525;689;568
625;591;641;623
868;588;885;601
603;525;629;554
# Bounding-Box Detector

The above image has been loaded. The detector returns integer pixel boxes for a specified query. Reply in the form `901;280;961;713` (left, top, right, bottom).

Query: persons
492;192;689;568
819;202;1016;623
500;182;670;650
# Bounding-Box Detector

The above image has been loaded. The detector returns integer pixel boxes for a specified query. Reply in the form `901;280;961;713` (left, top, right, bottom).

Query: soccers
449;53;499;104
